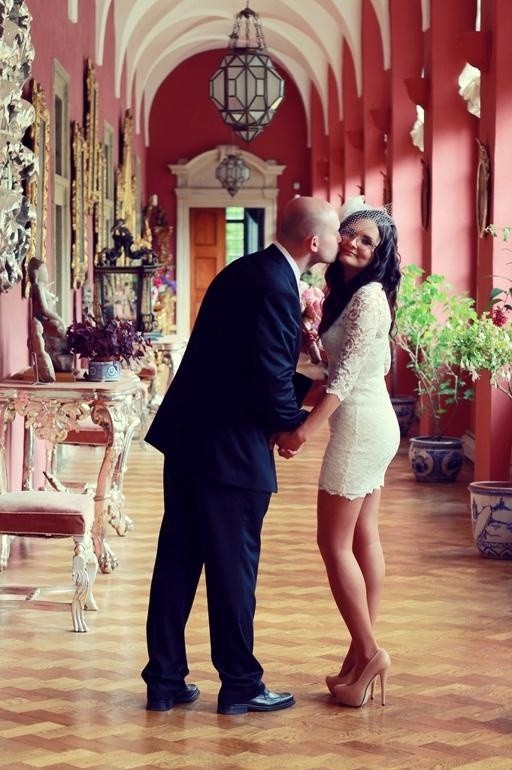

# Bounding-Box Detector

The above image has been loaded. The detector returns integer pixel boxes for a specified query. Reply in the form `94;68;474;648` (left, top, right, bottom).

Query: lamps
216;128;252;199
207;1;285;148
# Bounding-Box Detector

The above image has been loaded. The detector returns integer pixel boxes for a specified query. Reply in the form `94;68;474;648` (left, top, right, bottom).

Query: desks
156;331;188;415
1;369;150;577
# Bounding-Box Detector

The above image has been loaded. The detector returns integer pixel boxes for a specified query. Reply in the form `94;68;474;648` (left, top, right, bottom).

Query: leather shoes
216;686;295;715
145;683;200;711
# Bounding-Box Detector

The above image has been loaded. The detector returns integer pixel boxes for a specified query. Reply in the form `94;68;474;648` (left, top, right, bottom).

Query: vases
387;393;418;435
88;357;123;382
466;479;510;558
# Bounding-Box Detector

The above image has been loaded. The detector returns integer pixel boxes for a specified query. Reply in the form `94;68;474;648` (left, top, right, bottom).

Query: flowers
461;222;512;404
63;303;153;369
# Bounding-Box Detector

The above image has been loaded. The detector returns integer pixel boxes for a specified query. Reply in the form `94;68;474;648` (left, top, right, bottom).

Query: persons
23;257;71;354
266;193;402;710
135;192;345;717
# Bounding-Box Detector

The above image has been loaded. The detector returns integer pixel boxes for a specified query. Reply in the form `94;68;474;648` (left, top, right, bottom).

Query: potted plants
389;263;484;483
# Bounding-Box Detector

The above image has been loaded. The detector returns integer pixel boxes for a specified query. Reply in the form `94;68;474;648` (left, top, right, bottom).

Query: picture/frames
1;2;140;298
334;139;490;239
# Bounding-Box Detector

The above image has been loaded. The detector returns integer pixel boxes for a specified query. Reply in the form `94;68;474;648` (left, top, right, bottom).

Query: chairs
1;443;103;615
22;318;140;496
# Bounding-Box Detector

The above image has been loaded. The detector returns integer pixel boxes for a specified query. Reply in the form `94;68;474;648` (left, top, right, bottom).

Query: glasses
344;229;378;250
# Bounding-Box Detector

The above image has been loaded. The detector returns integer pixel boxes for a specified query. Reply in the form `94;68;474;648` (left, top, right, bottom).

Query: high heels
326;649;390;708
